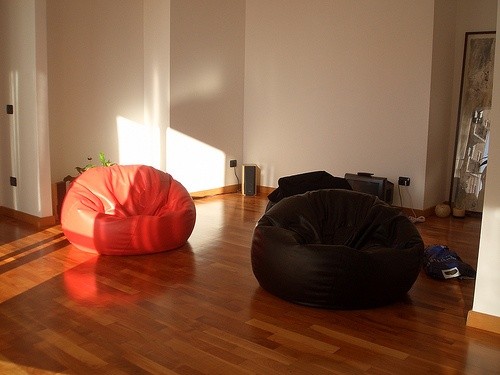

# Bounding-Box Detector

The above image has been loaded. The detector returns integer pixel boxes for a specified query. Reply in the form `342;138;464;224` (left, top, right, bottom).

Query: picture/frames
449;30;498;212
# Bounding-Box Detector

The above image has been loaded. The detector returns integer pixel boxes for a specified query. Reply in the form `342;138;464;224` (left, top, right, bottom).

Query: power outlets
229;159;238;167
398;176;411;187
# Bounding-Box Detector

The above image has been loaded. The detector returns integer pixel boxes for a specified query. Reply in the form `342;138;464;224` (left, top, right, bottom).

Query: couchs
265;170;352;217
250;188;425;310
59;164;197;257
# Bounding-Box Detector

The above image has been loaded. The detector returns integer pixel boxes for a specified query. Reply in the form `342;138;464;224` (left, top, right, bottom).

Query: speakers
241;164;260;196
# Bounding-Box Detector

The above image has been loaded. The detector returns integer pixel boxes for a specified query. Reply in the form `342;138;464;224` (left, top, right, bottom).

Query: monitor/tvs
344;172;394;206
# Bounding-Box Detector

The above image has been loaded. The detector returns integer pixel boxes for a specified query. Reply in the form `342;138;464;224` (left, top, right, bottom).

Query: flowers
63;151;119;188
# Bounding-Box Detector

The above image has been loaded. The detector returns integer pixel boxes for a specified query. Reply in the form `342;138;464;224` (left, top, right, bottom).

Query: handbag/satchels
419;244;477;279
